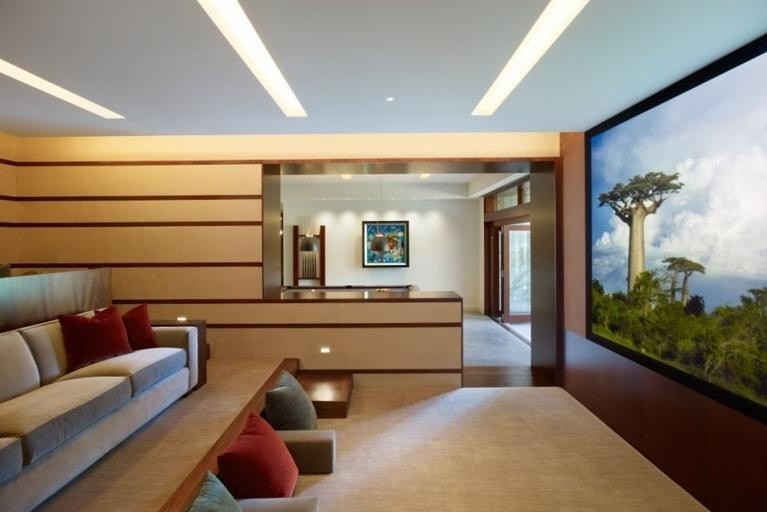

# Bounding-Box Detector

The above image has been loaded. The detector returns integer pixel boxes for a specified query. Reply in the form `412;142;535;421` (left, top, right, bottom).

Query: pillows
120;301;157;352
189;468;244;510
263;367;320;432
214;409;301;500
55;302;134;372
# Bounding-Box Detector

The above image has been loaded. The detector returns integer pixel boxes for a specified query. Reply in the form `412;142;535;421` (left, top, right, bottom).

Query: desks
148;316;208;393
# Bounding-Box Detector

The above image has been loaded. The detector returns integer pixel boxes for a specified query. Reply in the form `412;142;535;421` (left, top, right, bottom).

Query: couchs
0;263;199;512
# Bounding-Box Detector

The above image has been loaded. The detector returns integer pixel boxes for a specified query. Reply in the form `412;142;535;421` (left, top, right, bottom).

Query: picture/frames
360;221;411;270
582;31;766;424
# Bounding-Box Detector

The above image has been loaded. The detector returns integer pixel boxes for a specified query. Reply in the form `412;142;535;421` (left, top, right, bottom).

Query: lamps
367;173;392;256
297;233;321;252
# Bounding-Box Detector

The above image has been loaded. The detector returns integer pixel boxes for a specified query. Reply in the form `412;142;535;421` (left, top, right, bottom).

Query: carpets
291;386;716;512
